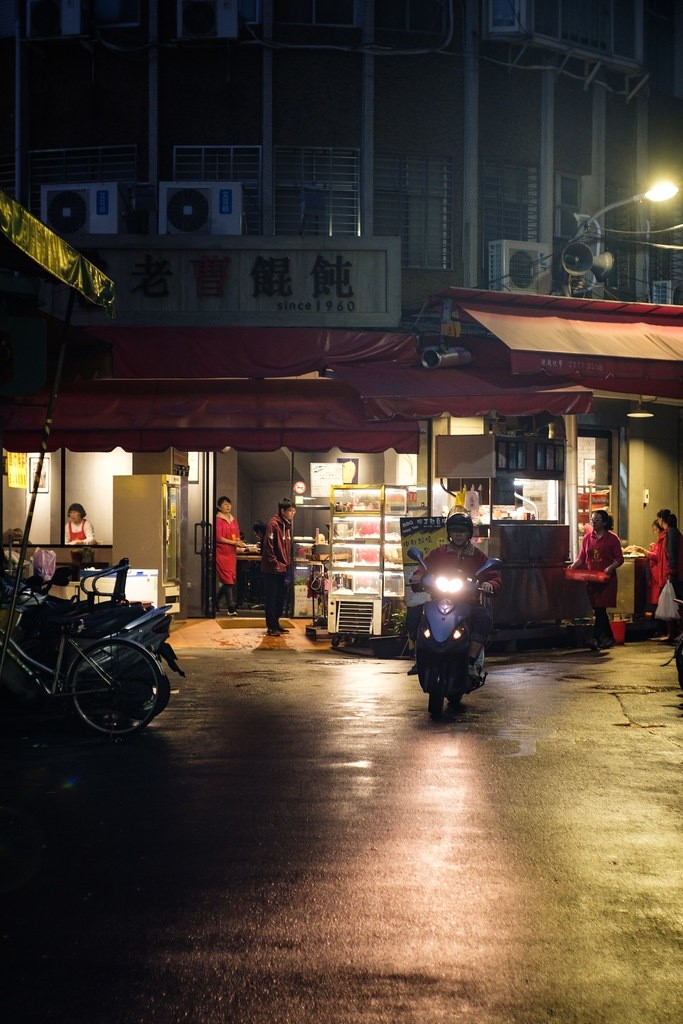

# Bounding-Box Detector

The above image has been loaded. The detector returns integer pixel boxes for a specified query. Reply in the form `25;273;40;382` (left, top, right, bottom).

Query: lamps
575;180;679;240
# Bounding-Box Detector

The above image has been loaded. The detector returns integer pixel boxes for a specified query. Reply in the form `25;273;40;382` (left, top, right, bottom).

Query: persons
213;496;246;616
567;509;624;650
63;503;97;545
635;509;682;643
407;513;501;678
234;519;267;605
261;497;297;636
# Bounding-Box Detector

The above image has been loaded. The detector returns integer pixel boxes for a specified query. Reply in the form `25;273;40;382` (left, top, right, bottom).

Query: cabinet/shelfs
329;484;410;599
578;483;611;538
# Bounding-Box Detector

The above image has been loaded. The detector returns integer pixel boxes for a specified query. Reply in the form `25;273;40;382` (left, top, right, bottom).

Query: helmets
445;512;474;539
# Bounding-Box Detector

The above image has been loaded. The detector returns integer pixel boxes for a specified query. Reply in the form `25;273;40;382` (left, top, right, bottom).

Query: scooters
405;545;504;715
0;558;186;725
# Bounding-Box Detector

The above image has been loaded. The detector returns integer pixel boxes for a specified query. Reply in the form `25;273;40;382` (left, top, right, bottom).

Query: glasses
284;509;296;513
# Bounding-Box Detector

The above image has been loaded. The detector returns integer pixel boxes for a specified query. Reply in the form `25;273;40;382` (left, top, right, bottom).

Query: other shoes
277;625;289;634
467;664;480;680
599;636;616;648
407;664;419;676
590;638;599;651
267;627;281;636
228;607;238;616
667;633;683;647
651;632;669;641
210;597;222;613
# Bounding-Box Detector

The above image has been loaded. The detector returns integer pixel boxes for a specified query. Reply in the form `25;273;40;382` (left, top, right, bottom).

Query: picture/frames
29;457;50;493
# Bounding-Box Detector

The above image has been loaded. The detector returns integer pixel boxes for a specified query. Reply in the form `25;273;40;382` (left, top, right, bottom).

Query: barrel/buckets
609;620;626;645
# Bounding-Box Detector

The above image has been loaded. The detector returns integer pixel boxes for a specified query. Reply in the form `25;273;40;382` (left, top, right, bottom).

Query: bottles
465;487;479;516
455;487;466;506
531;514;535;520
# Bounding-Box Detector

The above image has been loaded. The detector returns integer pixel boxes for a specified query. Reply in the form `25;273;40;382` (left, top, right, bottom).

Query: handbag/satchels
655;580;681;621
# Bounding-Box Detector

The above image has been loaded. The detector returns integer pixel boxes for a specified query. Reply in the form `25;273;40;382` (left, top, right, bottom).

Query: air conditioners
652;280;683;305
159;181;243;234
177;0;238;38
488;0;562;41
313;0;362;29
27;0;86;38
41;182;120;234
488;239;553;295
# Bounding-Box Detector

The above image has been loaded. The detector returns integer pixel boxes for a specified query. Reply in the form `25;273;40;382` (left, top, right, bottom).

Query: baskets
564;568;607;582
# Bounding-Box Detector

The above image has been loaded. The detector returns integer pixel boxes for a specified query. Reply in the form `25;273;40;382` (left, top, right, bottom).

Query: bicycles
0;580;163;742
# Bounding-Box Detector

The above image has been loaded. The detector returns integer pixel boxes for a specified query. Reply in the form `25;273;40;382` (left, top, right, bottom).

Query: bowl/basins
245;545;257;548
249;547;258;552
237;548;246;552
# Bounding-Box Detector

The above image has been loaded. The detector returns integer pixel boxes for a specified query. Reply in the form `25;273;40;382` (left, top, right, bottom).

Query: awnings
0;302;683;454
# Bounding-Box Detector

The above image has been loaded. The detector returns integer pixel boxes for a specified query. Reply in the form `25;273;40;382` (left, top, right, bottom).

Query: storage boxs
472;537;500;559
70;551;95;563
